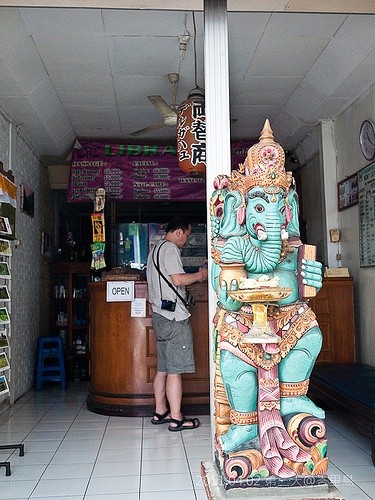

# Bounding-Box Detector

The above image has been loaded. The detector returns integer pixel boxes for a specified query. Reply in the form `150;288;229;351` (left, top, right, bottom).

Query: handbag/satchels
185;291;195;310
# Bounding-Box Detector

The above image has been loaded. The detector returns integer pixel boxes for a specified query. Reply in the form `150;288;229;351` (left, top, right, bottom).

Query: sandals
151;410;184;424
168;418;200;431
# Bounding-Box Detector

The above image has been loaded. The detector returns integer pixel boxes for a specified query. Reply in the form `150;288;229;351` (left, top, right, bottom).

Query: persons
146;218;208;432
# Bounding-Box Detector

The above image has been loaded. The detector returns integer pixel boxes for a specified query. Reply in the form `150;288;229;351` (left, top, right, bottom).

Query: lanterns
177;95;206;178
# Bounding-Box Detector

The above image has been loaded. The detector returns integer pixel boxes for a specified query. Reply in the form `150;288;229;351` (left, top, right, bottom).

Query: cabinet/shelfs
48;261;91;381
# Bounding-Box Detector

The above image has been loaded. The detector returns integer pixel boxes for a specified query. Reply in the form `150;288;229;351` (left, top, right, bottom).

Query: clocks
359;120;375;161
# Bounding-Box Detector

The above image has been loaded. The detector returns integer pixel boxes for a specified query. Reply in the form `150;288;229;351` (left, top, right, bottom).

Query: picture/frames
337;173;359;211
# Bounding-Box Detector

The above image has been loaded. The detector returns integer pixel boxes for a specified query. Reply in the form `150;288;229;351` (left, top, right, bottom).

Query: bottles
58;286;65;299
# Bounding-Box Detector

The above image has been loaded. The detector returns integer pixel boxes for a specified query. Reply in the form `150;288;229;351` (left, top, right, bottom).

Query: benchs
307;362;375;467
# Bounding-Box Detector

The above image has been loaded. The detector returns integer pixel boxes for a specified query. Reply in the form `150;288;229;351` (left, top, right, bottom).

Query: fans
127;73;238;135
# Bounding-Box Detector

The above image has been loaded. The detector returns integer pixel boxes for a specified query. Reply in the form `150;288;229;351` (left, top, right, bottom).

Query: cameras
161;299;176;312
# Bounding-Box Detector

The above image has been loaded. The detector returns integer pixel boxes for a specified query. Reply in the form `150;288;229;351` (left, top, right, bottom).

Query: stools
35;336;66;391
